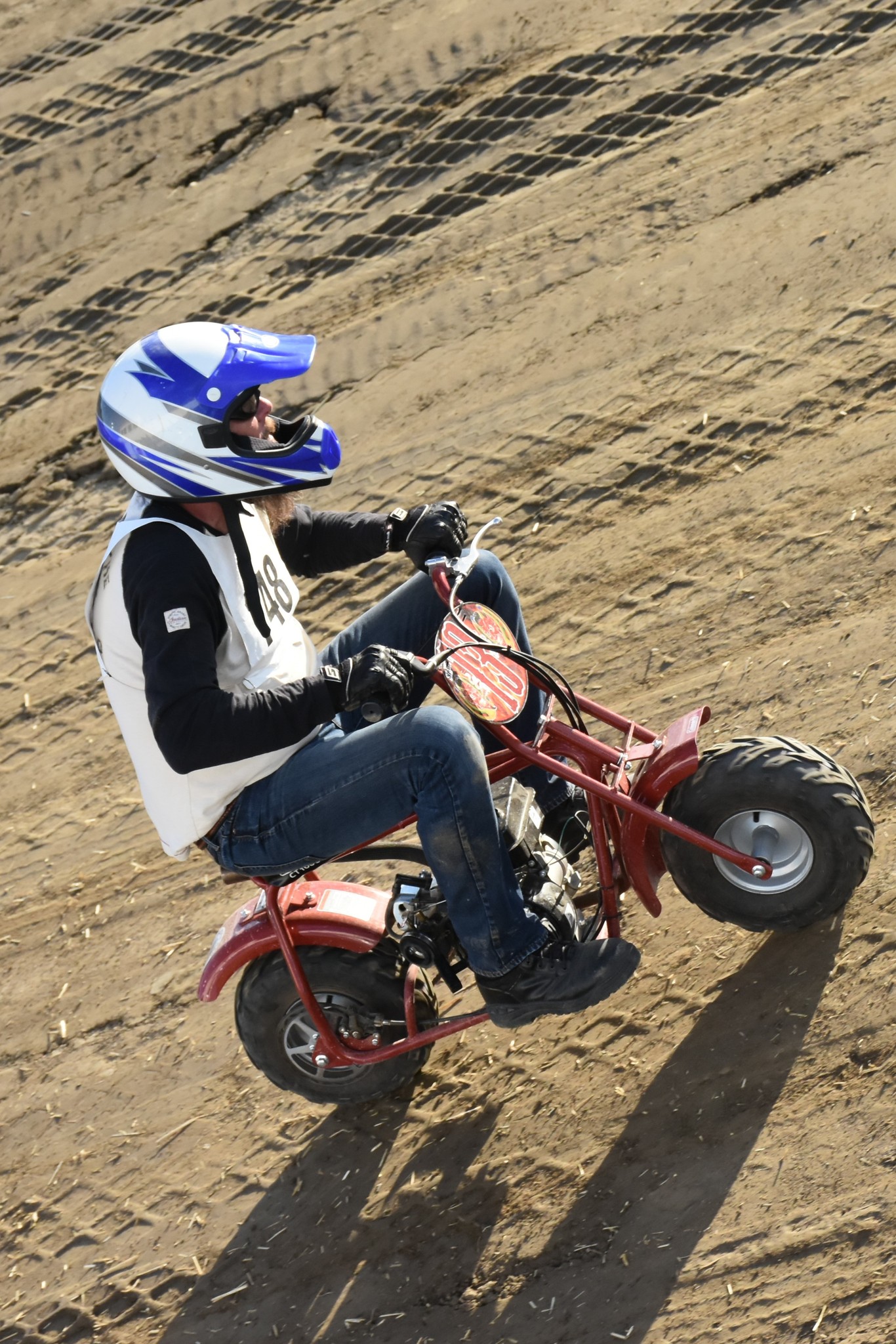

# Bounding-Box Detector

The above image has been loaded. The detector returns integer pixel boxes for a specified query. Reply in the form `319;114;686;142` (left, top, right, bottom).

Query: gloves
387;501;468;573
317;643;416;716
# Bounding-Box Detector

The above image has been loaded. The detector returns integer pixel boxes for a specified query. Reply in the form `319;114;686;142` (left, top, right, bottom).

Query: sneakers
474;938;641;1028
537;789;625;862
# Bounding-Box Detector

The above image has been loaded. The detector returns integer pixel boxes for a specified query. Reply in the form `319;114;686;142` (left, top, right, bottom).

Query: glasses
228;387;262;421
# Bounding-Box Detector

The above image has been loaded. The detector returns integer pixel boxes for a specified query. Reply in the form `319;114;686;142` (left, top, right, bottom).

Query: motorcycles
194;516;875;1106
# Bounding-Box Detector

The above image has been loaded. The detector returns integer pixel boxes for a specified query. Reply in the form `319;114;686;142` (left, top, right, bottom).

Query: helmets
96;321;341;501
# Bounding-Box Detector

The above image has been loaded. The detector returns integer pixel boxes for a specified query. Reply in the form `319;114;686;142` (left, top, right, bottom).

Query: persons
84;322;641;1031
